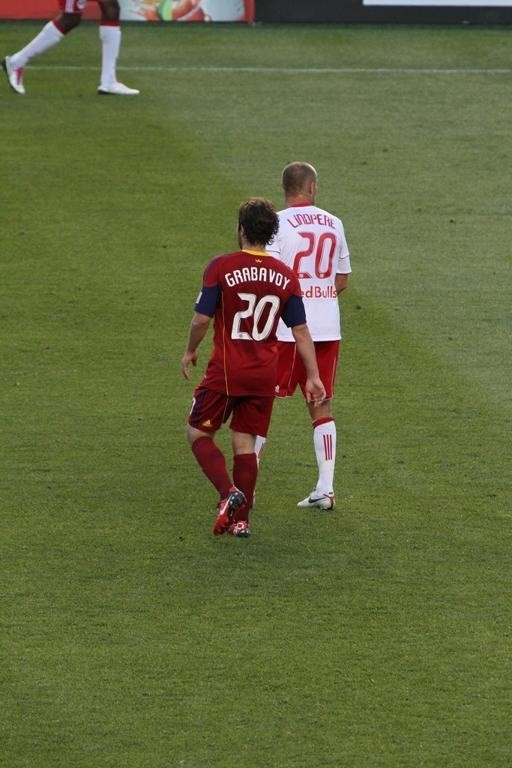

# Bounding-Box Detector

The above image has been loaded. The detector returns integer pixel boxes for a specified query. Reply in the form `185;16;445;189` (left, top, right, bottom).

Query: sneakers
2;55;26;96
212;484;247;537
97;83;141;96
296;490;336;510
228;520;252;538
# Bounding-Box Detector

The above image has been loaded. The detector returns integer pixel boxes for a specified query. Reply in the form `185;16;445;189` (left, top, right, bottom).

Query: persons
179;195;328;538
1;1;142;98
251;159;354;514
